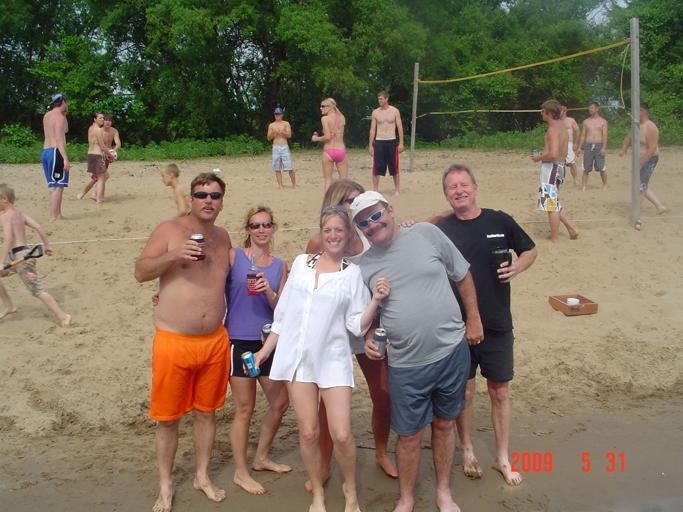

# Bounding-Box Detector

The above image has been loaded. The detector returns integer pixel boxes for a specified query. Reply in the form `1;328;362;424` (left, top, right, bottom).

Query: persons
311;97;348;194
558;105;581;189
75;114;120;204
618;102;670;217
366;90;404;198
1;181;73;328
305;177;457;493
149;203;294;497
160;161;193;219
265;105;297;190
574;102;612;194
532;98;581;244
37;93;76;221
85;112;106;204
399;164;541;486
348;190;483;512
133;171;231;511
238;202;393;512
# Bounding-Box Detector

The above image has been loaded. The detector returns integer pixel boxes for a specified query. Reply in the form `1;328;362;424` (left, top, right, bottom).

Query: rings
261;282;264;286
477;339;481;344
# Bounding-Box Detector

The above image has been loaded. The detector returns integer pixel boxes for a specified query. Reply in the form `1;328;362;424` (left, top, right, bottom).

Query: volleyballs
106;148;118;161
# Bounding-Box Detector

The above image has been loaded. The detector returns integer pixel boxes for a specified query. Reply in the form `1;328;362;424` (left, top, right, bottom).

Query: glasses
247;222;273;229
355;204;388;228
192;191;221;199
320;206;349;228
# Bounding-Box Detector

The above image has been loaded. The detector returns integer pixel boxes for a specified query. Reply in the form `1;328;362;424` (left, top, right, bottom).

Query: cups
247;270;262;296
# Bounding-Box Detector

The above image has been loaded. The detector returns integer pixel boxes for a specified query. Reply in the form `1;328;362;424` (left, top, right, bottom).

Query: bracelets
269;288;278;301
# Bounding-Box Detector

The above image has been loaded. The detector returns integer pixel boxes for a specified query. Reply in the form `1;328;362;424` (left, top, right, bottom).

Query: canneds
241;351;260;378
261;324;271;345
373;327;387;358
190;233;207;261
